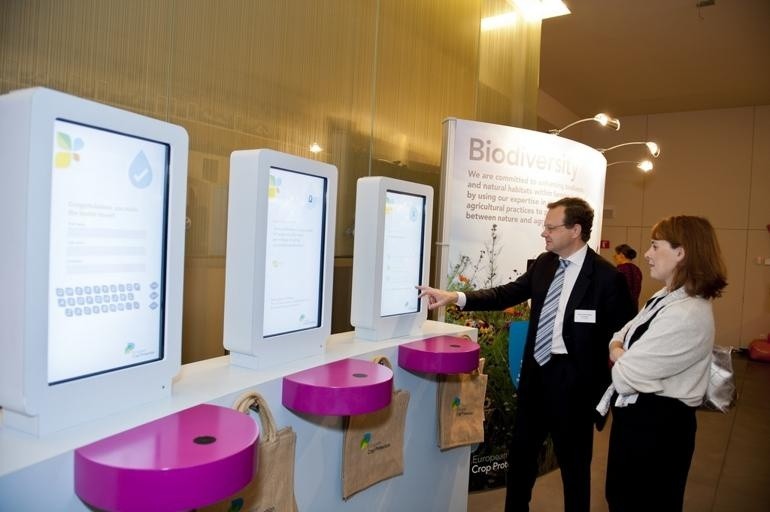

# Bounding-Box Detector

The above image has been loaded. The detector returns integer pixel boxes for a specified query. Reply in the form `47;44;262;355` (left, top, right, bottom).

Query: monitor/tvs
350;176;434;342
1;87;188;436
222;147;339;370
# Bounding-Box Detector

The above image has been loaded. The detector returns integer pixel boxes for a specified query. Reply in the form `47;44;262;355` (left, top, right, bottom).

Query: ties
534;258;571;366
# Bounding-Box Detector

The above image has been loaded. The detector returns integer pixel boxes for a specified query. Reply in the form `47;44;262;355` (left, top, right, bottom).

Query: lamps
541;113;620;136
598;142;660;159
607;160;653;172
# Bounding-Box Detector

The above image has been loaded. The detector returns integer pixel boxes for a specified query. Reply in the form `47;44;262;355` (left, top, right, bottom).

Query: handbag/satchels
438;334;488;450
342;357;409;497
702;345;738;415
196;393;299;511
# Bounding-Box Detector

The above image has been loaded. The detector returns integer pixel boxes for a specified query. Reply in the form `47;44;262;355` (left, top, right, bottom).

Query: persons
610;243;642;304
608;215;729;512
413;197;638;512
